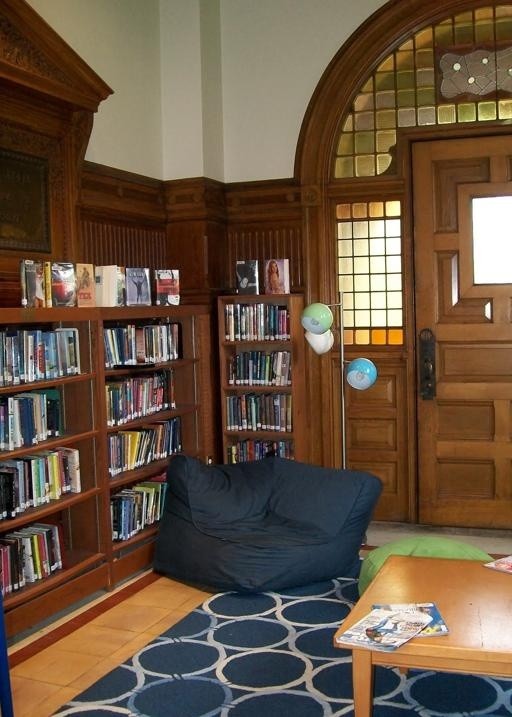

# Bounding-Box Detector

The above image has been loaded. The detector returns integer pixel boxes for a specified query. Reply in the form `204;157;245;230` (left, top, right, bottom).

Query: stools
357;535;496;596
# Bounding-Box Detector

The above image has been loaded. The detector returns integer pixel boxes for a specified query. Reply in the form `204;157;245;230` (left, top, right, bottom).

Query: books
336;609;433;653
482;552;512;576
370;602;450;639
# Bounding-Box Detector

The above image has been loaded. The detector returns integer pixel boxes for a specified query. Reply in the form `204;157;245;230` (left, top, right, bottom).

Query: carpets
50;557;512;717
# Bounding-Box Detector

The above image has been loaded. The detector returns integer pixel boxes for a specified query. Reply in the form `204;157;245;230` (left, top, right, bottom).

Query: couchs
152;454;383;596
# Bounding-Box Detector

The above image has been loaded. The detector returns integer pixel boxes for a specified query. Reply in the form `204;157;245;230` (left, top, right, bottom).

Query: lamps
300;291;378;469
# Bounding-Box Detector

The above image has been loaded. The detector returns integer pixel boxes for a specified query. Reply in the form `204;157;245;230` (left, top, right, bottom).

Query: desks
334;554;512;717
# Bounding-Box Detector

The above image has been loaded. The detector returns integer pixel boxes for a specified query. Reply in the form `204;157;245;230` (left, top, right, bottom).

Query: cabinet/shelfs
2;307;215;636
218;296;300;465
